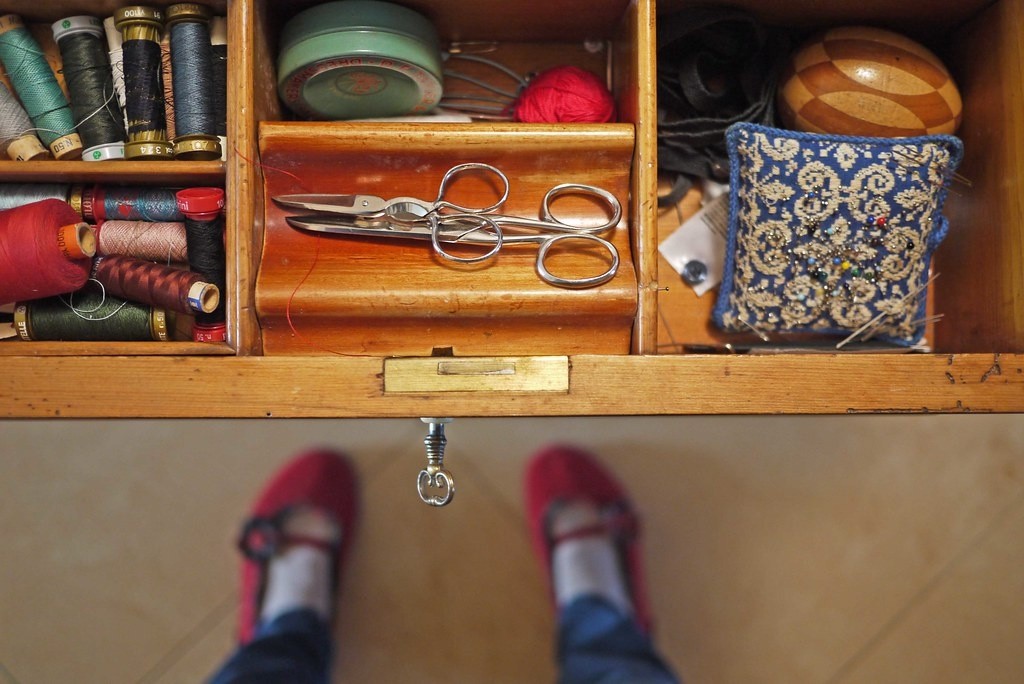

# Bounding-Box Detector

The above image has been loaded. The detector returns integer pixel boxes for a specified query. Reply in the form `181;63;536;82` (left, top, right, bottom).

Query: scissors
285;183;623;290
272;161;509;262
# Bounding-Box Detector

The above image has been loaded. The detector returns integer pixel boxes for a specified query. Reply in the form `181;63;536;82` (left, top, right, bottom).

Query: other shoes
235;448;363;669
526;446;648;647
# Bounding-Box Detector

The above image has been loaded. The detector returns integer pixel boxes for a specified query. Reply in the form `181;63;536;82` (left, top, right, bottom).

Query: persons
202;442;689;684
